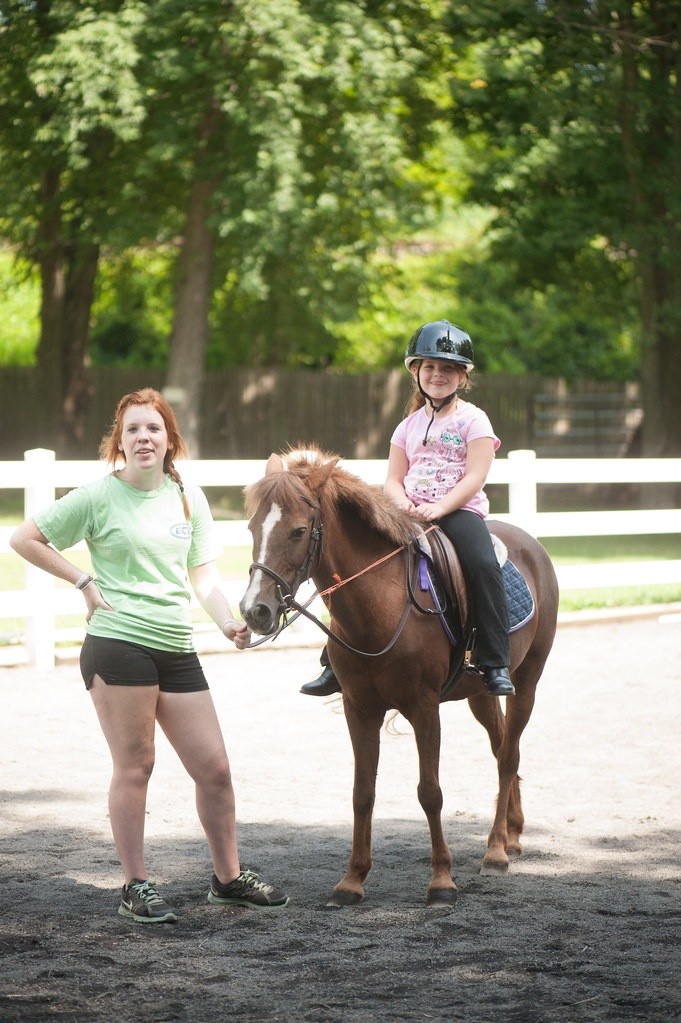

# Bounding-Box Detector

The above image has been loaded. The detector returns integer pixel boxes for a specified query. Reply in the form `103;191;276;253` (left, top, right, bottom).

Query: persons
296;318;517;697
11;389;290;923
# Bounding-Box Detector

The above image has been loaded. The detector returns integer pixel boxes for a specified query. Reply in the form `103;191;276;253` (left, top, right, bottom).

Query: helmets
404;319;474;372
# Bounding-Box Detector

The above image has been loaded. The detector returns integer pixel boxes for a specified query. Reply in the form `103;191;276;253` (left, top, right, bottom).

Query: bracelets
74;570;94;590
221;620;237;636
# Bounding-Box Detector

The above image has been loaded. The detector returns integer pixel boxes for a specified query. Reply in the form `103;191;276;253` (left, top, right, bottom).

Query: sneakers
207;865;289;909
117;879;177;922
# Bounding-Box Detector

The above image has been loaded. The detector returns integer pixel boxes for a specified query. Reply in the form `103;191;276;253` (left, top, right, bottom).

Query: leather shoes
298;666;342;696
483;666;515;696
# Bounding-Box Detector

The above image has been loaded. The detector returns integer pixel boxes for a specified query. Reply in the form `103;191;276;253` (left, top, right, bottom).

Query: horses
238;439;560;909
